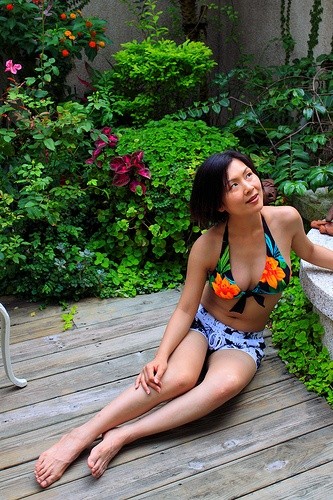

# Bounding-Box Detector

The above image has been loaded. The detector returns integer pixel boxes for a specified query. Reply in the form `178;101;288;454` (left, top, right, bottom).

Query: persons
31;149;333;488
